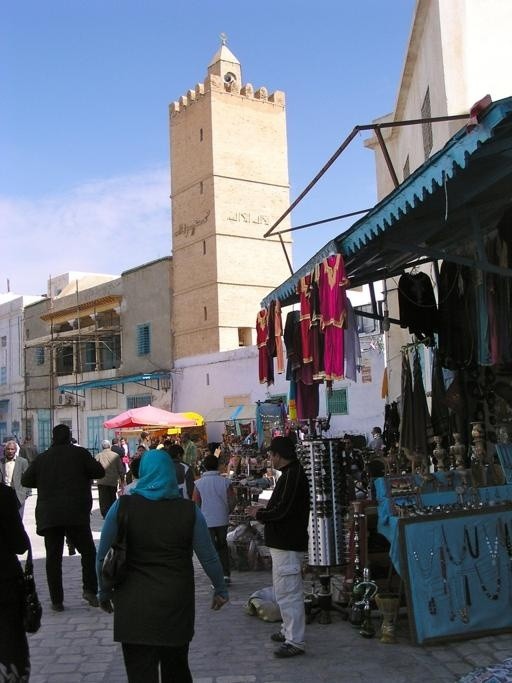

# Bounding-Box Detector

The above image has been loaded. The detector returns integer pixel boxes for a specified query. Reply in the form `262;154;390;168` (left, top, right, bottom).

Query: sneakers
82;589;100;608
51;601;64;611
270;631;286;643
272;642;306;658
211;576;231;590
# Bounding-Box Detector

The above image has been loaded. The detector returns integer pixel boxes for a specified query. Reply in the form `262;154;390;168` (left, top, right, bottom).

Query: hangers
302;244;347;278
409;264;420;275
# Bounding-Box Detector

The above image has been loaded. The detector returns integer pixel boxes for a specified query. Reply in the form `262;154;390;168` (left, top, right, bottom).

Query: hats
265;436;296;453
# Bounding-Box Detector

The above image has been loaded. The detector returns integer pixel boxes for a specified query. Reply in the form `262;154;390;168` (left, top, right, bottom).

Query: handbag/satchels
99;540;128;593
8;577;43;633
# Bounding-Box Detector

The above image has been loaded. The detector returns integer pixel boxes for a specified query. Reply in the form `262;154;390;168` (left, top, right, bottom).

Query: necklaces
409;515;511;626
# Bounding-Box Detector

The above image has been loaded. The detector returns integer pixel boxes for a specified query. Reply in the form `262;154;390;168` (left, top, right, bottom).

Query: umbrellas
101;403;198;433
166;411;204;435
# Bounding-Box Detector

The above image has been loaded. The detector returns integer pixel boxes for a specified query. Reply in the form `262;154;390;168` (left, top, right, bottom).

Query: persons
343;433;365;470
1;482;43;682
1;430;237;591
243;435;312;658
367;426;385;449
19;422;105;614
94;446;232;681
